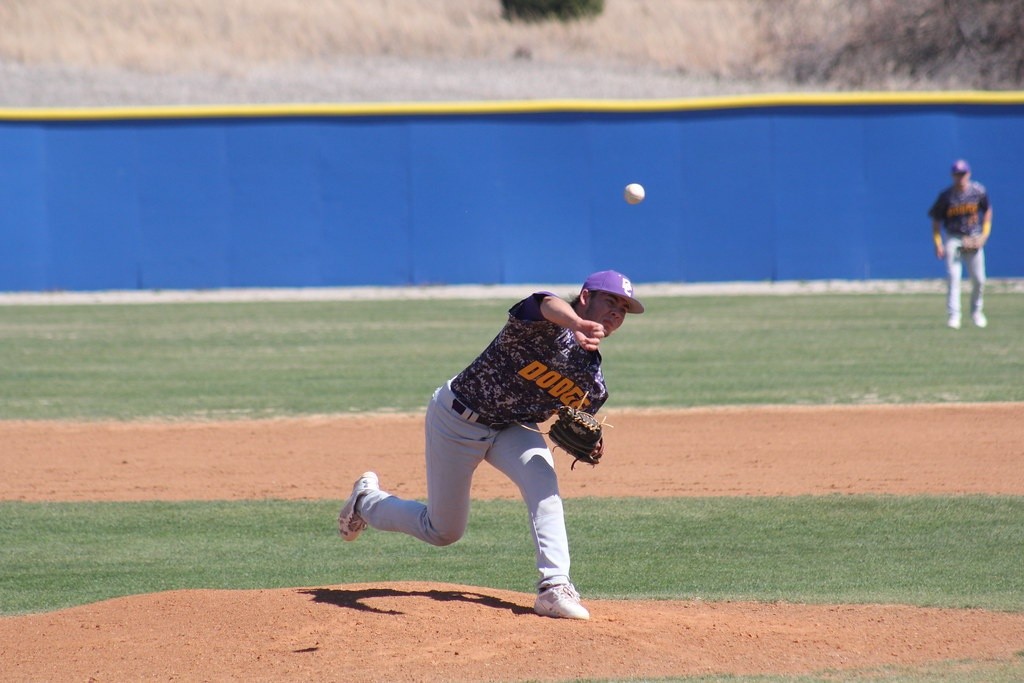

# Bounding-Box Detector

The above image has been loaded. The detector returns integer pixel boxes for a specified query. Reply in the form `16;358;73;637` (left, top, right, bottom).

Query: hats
582;269;644;314
951;160;969;175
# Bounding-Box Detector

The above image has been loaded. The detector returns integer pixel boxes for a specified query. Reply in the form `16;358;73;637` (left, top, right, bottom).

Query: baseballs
625;183;645;203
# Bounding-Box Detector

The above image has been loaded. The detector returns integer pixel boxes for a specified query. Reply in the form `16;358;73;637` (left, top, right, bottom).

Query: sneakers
338;471;379;541
533;583;589;621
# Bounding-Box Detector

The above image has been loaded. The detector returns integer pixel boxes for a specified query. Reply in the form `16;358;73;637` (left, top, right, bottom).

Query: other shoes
970;311;987;328
947;313;962;329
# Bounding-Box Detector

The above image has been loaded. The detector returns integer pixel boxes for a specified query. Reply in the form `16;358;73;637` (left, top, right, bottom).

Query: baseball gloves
548;404;604;463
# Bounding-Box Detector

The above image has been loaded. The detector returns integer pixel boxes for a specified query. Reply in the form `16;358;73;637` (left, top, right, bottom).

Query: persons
928;160;993;329
338;270;644;619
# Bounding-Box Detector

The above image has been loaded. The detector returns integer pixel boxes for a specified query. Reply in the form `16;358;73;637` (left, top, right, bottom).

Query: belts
451;400;509;430
